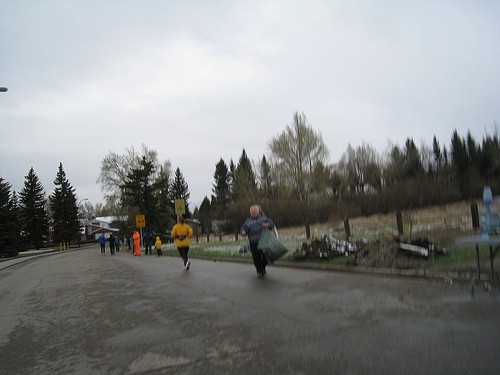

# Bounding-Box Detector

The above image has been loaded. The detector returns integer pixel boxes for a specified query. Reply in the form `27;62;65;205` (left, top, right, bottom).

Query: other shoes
184;262;191;271
256;270;267;277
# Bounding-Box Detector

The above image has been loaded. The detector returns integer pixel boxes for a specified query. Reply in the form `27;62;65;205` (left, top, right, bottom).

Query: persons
241;204;274;278
142;230;163;256
171;216;193;273
133;229;141;256
99;232;120;256
